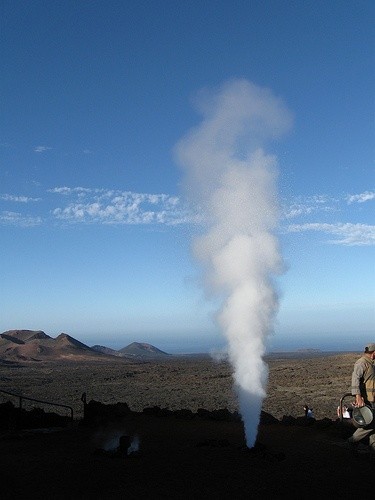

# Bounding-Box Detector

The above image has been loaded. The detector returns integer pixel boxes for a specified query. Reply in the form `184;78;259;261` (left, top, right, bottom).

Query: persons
304;406;313;418
347;341;375;454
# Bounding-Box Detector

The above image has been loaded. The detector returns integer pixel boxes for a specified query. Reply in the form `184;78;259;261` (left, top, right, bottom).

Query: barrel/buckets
352;404;374;429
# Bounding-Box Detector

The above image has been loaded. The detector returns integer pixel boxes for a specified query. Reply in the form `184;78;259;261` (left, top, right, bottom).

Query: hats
364;343;375;353
